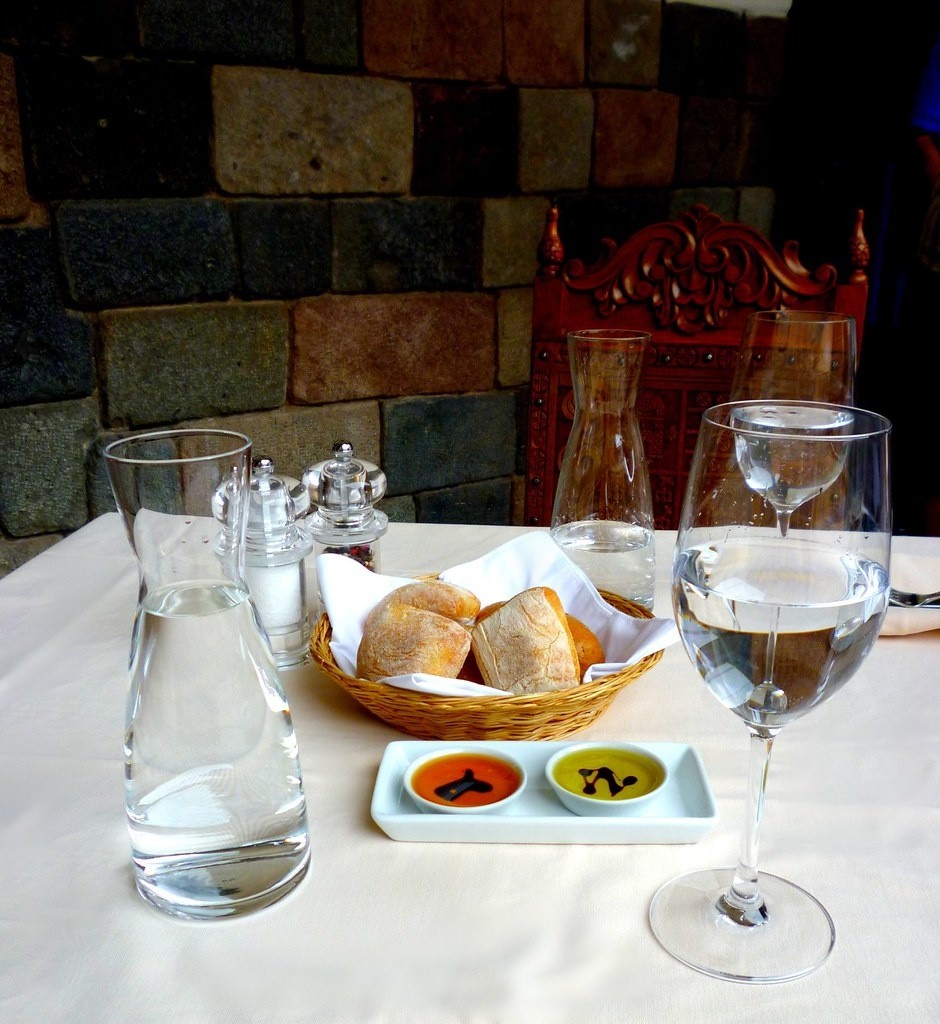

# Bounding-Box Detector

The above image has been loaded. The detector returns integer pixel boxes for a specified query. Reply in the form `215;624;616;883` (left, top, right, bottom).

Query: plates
371;737;719;843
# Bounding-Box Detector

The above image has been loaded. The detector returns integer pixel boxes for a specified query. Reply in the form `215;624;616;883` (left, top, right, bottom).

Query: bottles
209;454;312;672
302;441;389;604
105;429;312;919
552;330;656;613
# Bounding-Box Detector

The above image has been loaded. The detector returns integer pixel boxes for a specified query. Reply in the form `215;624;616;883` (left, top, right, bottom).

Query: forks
890;588;939;607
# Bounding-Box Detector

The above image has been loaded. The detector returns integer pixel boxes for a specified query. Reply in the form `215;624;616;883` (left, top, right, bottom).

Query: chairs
525;206;869;530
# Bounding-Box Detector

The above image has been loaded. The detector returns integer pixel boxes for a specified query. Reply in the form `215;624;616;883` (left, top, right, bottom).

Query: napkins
865;549;940;638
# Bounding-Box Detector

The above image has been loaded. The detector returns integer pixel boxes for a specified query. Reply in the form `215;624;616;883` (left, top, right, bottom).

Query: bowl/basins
545;742;671;817
404;749;525;815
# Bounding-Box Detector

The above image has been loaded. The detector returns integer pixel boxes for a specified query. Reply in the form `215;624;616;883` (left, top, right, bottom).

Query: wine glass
728;309;856;538
645;400;892;982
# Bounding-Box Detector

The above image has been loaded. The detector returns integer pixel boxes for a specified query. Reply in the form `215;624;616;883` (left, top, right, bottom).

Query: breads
354;580;604;697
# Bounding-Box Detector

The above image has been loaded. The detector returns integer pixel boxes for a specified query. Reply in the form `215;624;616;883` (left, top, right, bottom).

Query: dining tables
0;512;940;1024
